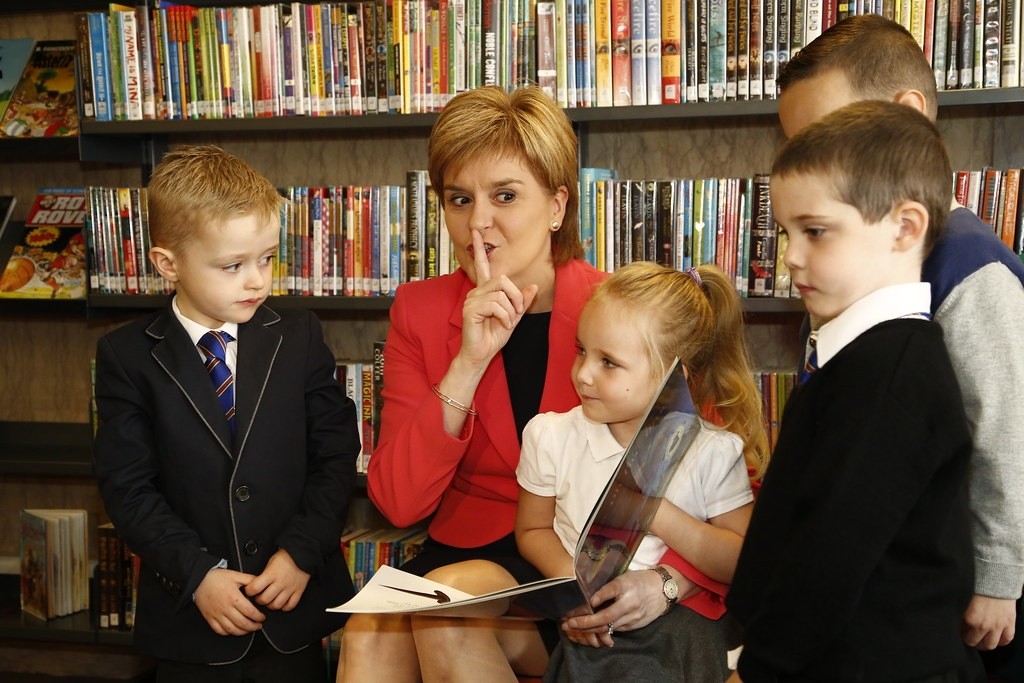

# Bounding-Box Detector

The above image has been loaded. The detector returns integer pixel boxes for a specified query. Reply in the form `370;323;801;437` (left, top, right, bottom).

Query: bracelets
432;384;478;417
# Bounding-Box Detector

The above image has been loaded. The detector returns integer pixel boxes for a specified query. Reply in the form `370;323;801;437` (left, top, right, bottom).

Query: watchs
650;566;680;615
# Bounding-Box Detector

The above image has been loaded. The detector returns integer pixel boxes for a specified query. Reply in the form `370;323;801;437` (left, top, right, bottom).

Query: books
753;367;797;441
341;528;428;592
325;354;700;622
19;507;140;628
1;0;1024;300
334;342;389;471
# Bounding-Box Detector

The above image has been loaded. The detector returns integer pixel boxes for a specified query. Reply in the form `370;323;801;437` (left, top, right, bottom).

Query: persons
334;84;762;683
513;260;772;682
726;101;971;683
778;15;1024;649
93;143;362;683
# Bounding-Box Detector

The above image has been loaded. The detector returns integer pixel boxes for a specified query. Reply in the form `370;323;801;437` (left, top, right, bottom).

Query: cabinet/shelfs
0;0;1024;683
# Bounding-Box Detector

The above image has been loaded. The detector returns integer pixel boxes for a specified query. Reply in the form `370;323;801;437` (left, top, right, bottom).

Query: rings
608;623;615;635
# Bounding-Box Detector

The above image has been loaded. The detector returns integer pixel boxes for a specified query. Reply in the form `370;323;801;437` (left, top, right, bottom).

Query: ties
197;331;240;445
